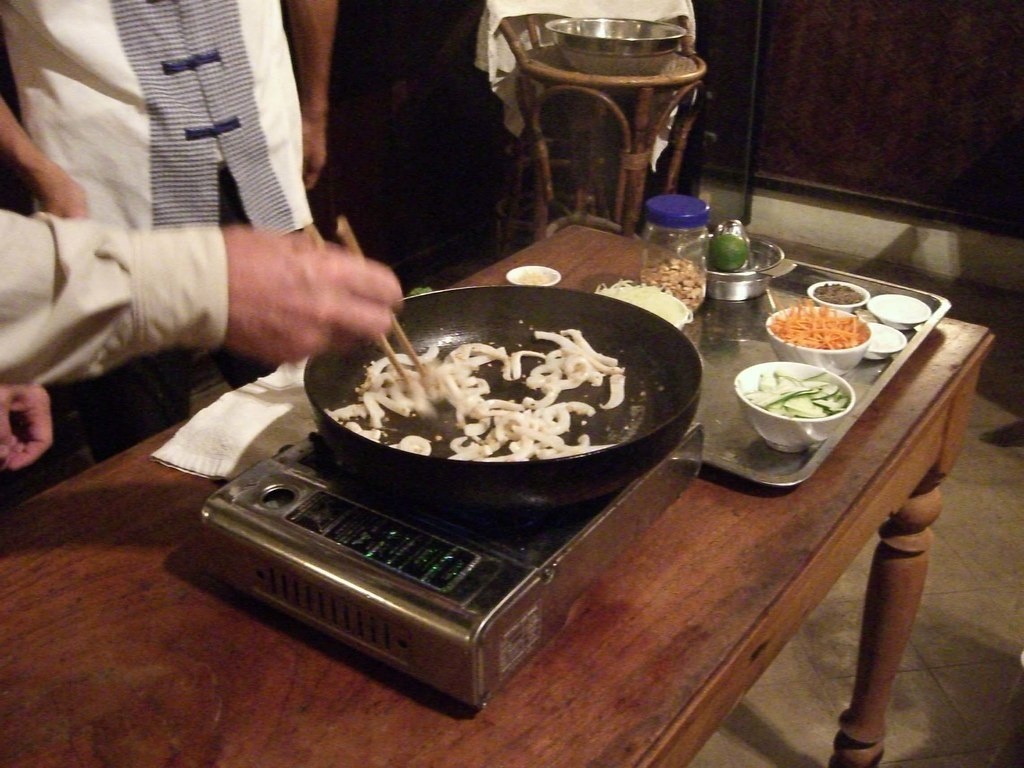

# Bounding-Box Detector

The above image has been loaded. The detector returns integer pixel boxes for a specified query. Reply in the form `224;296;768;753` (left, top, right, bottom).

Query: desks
0;219;988;768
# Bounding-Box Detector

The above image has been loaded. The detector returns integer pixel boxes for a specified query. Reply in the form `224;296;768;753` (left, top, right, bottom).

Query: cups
504;265;562;286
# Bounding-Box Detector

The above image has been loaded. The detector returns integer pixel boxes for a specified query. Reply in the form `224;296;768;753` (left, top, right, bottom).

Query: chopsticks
303;215;425;384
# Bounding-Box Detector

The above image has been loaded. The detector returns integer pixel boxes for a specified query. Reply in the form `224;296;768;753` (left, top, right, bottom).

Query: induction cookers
199;421;704;717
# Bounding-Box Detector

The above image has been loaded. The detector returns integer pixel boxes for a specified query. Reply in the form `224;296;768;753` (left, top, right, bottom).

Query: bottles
638;193;711;313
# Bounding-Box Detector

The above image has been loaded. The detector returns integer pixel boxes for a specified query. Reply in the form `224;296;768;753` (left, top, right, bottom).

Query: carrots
768;298;871;351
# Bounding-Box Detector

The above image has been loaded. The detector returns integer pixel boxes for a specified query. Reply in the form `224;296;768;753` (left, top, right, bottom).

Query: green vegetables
409;287;435;296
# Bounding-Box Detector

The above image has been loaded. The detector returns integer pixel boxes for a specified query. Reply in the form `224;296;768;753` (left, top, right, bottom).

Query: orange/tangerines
703;231;746;272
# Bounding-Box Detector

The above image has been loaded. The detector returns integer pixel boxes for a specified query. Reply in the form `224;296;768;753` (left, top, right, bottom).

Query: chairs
475;0;707;240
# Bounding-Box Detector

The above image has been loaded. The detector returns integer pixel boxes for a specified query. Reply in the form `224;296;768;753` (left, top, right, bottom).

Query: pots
678;237;798;302
302;284;704;506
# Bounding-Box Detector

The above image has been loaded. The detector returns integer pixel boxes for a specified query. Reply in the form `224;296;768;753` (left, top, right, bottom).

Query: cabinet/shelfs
691;0;1024;242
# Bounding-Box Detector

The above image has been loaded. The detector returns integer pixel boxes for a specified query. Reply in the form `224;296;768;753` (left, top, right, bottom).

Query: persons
1;0;341;465
0;206;405;474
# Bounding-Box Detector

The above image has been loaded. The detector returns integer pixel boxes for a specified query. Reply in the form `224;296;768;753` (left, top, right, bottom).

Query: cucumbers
740;370;847;419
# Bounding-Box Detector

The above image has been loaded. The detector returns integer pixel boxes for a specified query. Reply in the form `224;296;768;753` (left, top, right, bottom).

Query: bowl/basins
593;287;695;334
734;361;856;452
765;305;872;376
863;322;908;360
807;280;871;313
867;293;932;330
545;16;687;76
